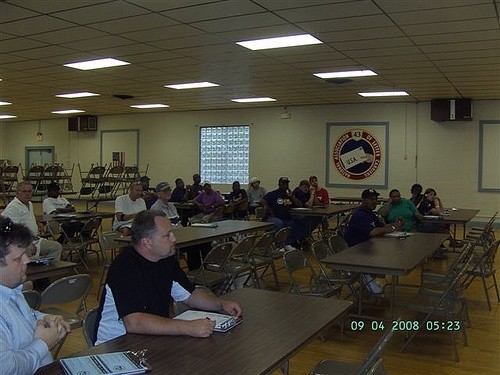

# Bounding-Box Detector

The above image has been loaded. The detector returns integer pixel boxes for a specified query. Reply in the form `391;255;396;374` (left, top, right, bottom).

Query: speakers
431;99;473;121
68;116;97;131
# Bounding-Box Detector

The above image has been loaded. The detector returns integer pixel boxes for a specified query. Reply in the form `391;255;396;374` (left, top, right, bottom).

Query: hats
249;177;260;184
279;177;290;184
156;182;169;191
362;189;380;198
202;180;211;187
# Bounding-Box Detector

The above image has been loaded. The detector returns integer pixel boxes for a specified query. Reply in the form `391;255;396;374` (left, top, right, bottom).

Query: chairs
0;162;500;375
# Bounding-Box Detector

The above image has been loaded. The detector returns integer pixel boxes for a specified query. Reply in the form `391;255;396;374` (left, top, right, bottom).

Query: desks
421;209;480;254
115;220;276;260
290;204;358;236
320;233;451;329
26;261;78;282
34;289;354;375
37;212;115;224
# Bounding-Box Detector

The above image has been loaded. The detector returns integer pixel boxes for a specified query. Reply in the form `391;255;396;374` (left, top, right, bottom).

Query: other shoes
434;252;448;260
438;244;448;251
449;241;464;248
275;245;296;251
362;277;384;294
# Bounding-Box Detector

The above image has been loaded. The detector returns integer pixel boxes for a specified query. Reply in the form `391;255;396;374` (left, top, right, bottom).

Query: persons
408;184;464;248
111;176;212;271
0;182;62;260
266;176;329;251
228;179;265;219
345;190;401;294
379;189;448;258
93;211;241;346
43;182;83;244
0;216;71;375
170;174;224;228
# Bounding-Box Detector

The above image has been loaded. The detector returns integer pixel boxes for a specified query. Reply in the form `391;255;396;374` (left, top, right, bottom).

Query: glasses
163;189;172;192
0;217;13;236
